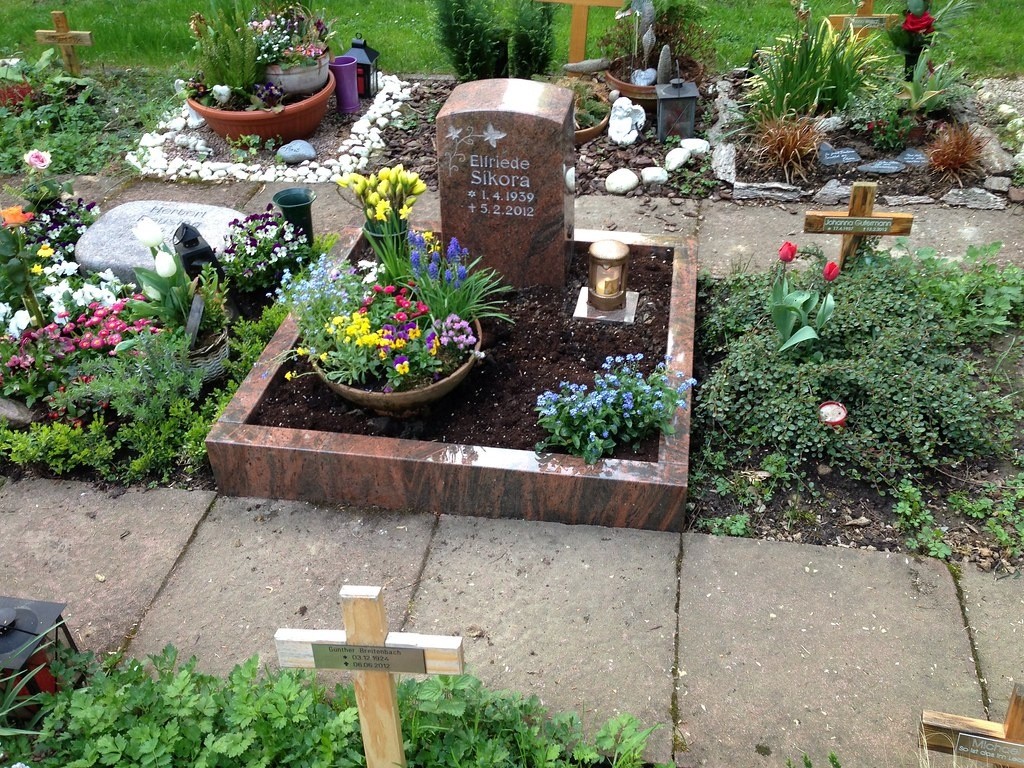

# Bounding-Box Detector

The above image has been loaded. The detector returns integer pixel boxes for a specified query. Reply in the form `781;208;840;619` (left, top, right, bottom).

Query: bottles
271;188;317;248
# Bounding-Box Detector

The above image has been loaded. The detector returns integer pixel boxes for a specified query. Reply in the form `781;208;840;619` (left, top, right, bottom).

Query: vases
363;220;409;266
605;52;703;111
328;56;361;116
265;44;331;97
573;92;611;145
902;41;932;82
306;312;483;416
897;122;928;148
186;70;337;152
273;187;318;250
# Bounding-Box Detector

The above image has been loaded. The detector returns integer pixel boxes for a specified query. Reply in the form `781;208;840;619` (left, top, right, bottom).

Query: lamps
588;240;628;312
172;223;226;293
655;60;700;144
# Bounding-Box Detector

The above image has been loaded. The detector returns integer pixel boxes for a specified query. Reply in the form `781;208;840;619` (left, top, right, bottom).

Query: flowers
1;164;672;447
891;5;940;52
776;238;844;349
865;118;905;144
171;2;327;109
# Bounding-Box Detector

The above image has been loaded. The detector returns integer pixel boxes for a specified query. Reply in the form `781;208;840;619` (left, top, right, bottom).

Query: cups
329;56;360;114
815;400;848;428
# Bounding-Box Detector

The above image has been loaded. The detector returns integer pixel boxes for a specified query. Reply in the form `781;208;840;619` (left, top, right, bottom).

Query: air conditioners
346;33;381;99
